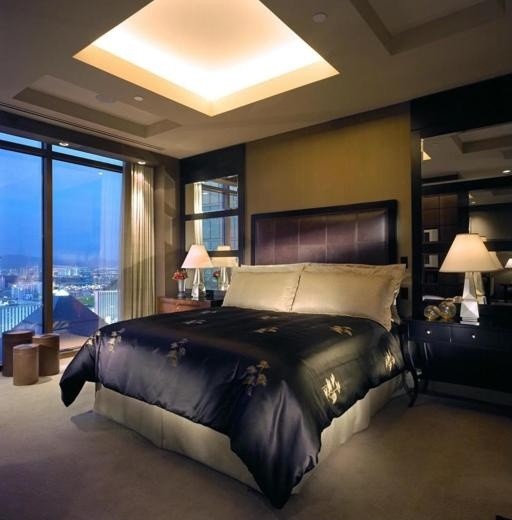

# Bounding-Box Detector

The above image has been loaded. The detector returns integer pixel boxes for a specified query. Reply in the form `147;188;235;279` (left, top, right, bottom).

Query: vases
176;279;185;297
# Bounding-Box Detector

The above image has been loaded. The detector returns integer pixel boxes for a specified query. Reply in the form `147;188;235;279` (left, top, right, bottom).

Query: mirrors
419;120;512;307
181;174;238;287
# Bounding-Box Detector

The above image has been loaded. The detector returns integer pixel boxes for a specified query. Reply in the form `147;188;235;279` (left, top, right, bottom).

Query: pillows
220;261;407;333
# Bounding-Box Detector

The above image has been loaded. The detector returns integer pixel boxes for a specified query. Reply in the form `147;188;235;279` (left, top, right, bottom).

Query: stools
2;327;60;388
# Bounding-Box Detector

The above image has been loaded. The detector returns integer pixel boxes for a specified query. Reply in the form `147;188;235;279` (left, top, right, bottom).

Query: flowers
171;270;187;281
212;271;219;279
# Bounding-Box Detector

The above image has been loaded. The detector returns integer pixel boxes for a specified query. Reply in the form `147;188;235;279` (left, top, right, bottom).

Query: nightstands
405;299;512;419
156;289;225;319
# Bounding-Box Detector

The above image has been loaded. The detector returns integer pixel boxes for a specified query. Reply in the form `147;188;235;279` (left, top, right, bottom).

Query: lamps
211;246;237;290
437;233;504;329
474;237;504;304
178;243;214;300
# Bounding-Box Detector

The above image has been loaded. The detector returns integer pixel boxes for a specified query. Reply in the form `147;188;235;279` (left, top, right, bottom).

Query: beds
90;263;420;510
